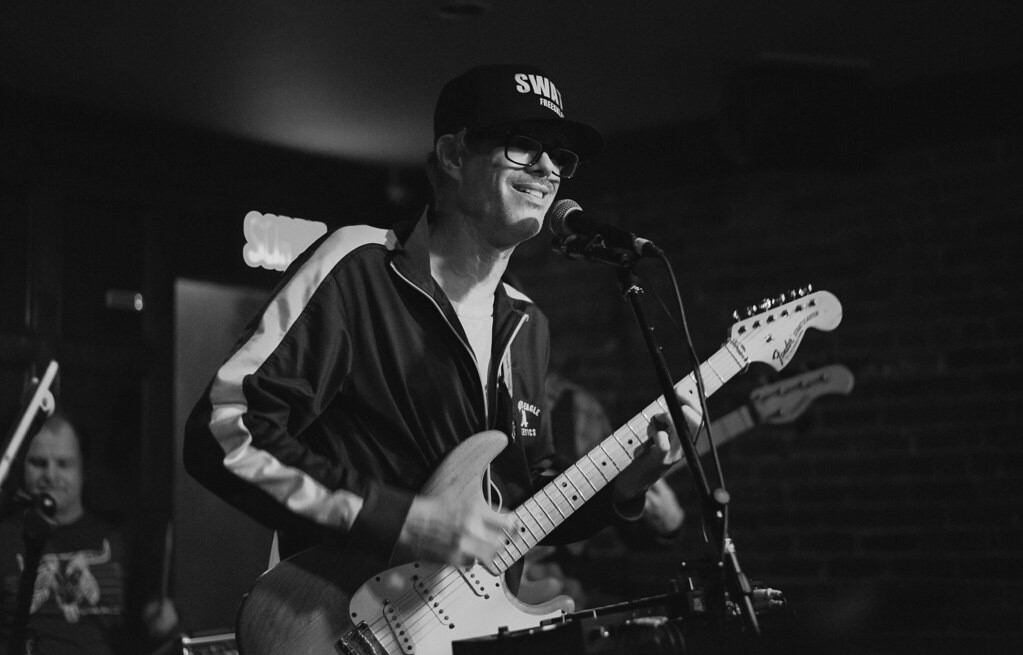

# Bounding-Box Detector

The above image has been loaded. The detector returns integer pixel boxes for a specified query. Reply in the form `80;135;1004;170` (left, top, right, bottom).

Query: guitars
661;364;856;475
238;284;849;655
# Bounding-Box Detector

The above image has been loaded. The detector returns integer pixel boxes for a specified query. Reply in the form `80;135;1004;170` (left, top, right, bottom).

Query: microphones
547;198;663;258
16;492;58;519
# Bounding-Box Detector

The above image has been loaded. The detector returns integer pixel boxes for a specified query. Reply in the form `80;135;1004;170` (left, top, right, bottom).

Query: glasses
468;127;580;179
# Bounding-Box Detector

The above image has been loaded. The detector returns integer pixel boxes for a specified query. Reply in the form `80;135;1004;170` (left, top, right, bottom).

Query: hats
432;64;603;159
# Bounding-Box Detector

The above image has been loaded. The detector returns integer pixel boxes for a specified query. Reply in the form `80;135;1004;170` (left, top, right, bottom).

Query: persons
0;402;179;655
183;63;703;655
